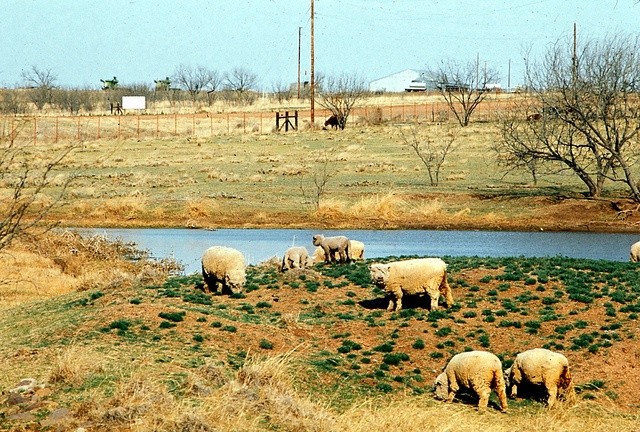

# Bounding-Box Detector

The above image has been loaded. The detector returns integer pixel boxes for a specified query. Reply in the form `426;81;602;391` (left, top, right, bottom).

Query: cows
325;114;344;130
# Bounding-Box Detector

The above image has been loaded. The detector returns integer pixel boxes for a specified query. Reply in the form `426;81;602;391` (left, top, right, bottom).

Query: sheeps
504;348;577;408
434;351;509;415
312;246;331;264
281;247;316;270
312;235;350;263
201;246;247;294
367;258;454;312
629;241;640;262
335;240;364;260
